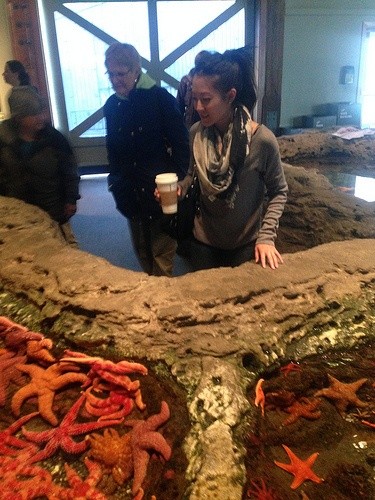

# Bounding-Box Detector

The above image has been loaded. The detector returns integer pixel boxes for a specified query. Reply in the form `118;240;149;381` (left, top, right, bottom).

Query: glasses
105;69;132;79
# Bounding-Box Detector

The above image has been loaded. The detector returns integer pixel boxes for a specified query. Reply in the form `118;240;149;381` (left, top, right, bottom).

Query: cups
155;172;178;214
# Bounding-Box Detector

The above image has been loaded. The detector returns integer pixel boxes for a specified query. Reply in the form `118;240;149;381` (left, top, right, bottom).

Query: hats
8;86;42;117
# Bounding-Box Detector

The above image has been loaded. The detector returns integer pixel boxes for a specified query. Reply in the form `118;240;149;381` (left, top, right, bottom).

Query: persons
154;47;289;274
0;61;81;239
104;44;190;277
176;51;211;131
